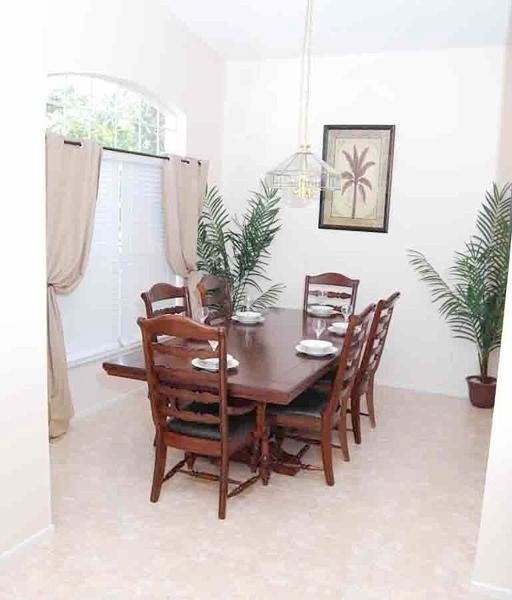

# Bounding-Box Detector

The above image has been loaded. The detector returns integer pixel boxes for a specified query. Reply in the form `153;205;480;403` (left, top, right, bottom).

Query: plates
192;357;240;372
307;309;339;317
231;316;266;323
328;325;347;335
295;345;338;357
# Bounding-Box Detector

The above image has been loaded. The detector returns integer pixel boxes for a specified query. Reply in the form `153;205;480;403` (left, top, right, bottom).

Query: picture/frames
319;124;394;233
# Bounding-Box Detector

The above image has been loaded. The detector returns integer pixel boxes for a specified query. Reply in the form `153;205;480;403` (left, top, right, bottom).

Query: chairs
198;272;232;329
278;302;376;486
303;270;358;325
142;282;191;348
138;315;270;519
318;292;401;442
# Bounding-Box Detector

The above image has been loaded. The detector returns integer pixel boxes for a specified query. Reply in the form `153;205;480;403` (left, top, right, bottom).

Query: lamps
266;0;345;202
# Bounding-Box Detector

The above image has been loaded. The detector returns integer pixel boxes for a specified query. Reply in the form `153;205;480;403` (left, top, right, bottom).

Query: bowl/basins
300;339;332;352
311;306;334;312
332;323;348;330
236;312;262;319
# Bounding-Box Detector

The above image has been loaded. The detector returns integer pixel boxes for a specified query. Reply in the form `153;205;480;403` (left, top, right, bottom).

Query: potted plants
406;180;512;409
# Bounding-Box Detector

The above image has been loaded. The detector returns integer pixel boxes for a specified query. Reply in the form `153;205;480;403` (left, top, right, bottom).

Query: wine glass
318;285;328;305
341;303;352;322
190;307;209;323
312;319;326;339
244;295;253;311
